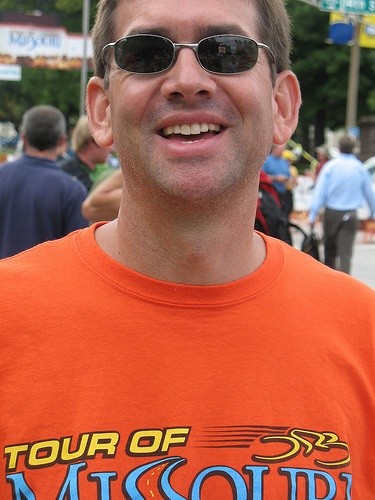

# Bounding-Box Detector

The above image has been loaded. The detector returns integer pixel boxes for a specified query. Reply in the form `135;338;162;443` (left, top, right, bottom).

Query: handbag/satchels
302;233;319;261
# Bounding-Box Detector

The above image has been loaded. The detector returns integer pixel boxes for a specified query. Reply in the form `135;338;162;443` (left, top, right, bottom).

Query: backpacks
252;181;288;242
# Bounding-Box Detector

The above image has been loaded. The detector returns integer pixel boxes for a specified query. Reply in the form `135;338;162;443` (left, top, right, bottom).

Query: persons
308;132;375;276
0;106;89;262
0;0;374;500
0;106;375;280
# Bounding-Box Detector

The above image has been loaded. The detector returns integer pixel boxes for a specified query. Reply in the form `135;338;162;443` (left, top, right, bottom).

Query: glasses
102;34;275;75
